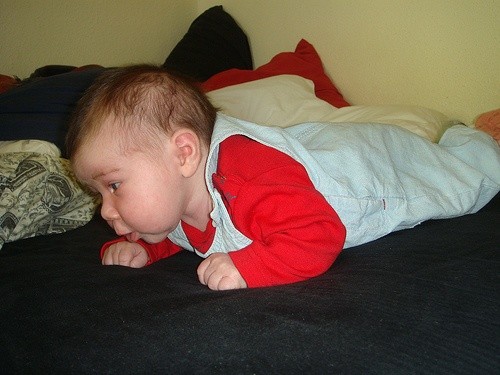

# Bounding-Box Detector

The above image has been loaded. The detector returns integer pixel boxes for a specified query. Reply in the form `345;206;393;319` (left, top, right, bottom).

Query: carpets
0;194;500;375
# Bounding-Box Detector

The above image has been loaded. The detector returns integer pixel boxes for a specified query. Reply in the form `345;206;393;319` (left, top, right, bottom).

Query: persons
65;64;500;292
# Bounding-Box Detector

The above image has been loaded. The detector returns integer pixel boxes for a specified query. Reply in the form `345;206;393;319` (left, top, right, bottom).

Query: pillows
201;73;458;146
191;38;352;108
